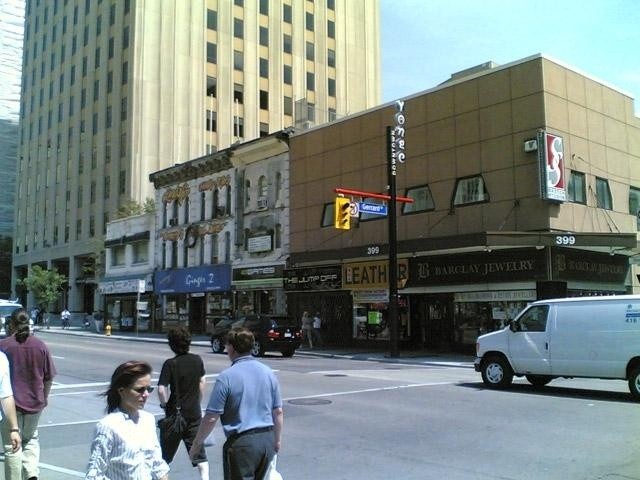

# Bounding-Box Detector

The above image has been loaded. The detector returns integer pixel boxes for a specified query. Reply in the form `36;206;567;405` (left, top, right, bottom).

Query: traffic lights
335;197;351;230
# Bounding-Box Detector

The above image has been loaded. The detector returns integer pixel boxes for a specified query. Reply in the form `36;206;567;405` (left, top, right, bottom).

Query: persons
0;317;23;454
86;359;172;480
0;308;58;480
30;305;104;335
4;315;17;336
189;326;284;479
156;326;211;480
298;311;316;350
312;312;325;347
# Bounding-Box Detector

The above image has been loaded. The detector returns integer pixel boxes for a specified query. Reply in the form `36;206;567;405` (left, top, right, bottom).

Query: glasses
130;387;154;393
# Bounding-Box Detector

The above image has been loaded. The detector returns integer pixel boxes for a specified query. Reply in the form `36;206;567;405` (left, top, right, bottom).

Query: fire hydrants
106;324;112;336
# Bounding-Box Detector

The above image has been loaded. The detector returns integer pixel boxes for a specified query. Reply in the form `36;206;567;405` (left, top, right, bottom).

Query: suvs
211;313;303;357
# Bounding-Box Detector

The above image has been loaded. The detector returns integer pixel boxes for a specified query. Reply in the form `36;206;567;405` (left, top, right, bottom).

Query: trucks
473;293;640;403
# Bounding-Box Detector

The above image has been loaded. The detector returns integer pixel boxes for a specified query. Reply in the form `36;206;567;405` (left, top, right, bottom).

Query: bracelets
191;440;203;448
159;403;166;410
8;427;21;433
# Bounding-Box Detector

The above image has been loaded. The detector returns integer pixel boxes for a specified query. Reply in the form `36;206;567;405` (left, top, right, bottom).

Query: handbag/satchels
157;406;188;443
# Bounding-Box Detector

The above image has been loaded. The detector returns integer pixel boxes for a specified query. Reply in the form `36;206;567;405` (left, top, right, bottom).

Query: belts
238;426;272;436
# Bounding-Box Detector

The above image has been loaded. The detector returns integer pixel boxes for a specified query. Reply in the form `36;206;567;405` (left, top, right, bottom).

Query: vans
0;299;35;340
